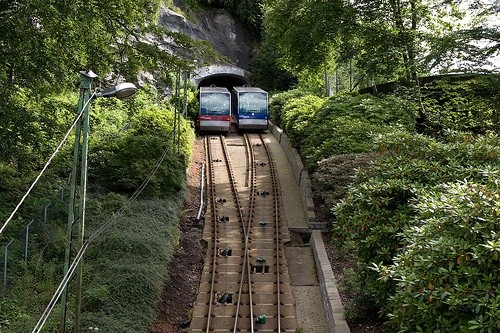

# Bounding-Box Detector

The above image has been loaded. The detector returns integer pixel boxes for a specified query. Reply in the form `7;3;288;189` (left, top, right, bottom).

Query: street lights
74;82;138;332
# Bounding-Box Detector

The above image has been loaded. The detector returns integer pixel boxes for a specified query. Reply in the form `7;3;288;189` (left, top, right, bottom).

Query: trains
231;85;269;130
198;85;231;134
